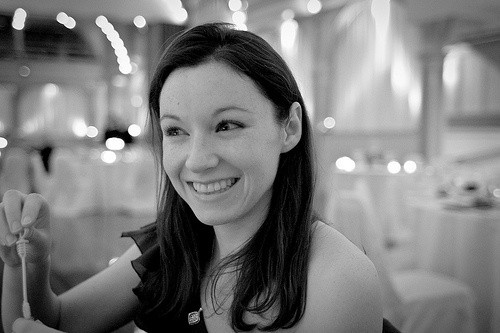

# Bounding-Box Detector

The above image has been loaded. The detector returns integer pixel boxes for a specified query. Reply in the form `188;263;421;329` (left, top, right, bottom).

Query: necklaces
186;226;253;326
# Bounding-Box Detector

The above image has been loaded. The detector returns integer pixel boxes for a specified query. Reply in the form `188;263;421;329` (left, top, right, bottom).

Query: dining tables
405;197;500;333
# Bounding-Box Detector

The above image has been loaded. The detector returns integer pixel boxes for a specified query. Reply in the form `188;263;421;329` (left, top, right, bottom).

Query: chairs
324;190;478;333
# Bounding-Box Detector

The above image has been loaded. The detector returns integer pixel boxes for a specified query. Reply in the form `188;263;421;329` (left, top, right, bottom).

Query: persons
0;21;384;333
